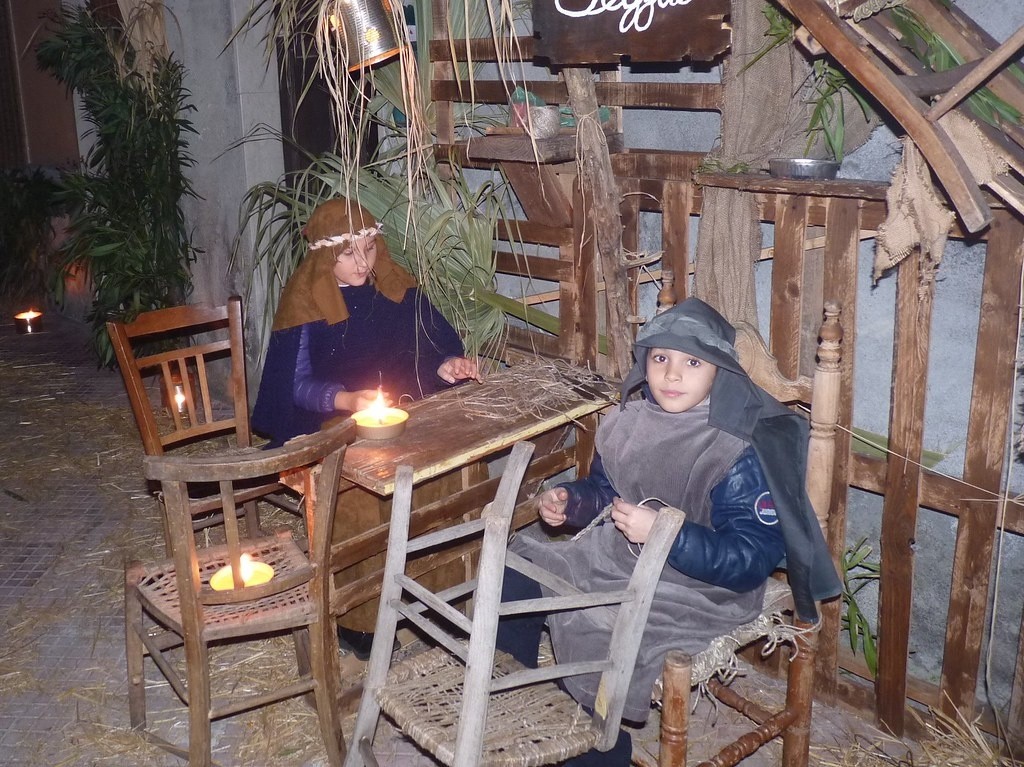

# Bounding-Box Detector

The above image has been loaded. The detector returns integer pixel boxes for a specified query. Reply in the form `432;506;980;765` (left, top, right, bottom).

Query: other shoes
338;620;402;661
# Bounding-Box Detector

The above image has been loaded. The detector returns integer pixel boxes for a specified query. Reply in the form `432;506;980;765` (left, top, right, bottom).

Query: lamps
325;0;409;73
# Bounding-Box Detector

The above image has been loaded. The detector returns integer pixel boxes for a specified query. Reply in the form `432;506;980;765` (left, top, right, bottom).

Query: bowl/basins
769;157;839;180
350;408;409;440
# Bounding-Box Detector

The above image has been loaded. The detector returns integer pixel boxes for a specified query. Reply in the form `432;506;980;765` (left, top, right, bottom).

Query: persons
250;199;484;660
496;297;844;767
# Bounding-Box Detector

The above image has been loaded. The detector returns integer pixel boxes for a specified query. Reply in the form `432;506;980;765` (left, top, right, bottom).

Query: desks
319;356;621;650
293;356;621;718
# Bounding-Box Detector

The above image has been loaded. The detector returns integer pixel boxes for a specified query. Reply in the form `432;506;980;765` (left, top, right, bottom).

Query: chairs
543;298;844;767
340;437;686;767
106;294;312;568
124;416;357;767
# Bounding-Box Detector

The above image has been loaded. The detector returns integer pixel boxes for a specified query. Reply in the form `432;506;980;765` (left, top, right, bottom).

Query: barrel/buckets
158;373;198;414
